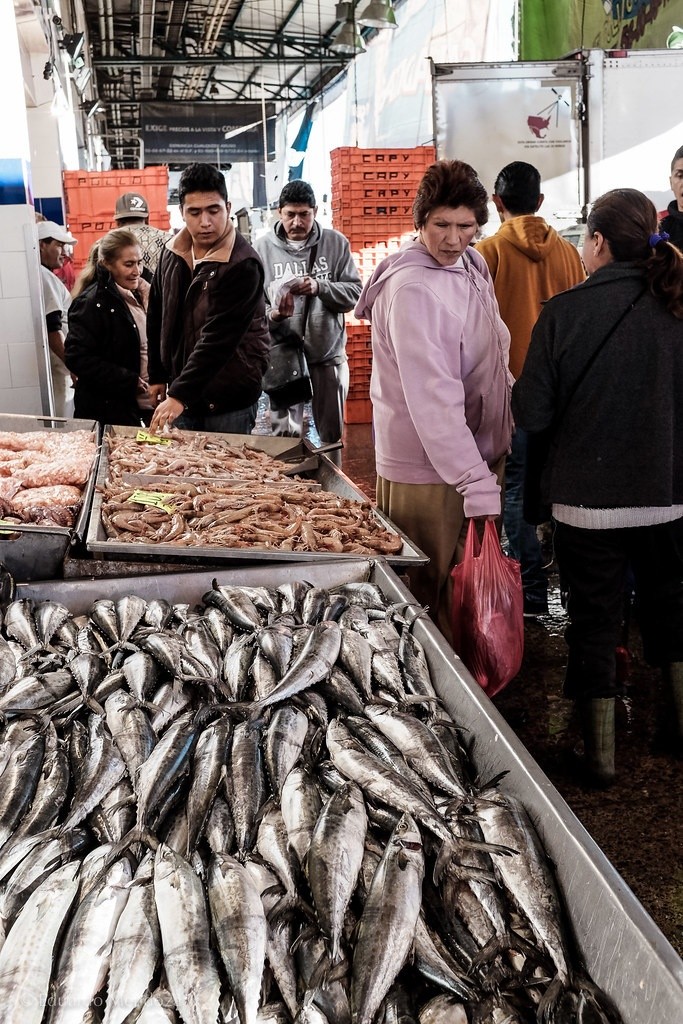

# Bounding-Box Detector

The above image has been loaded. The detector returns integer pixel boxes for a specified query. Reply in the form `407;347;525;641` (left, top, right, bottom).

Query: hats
113;192;149;219
38;221;77;245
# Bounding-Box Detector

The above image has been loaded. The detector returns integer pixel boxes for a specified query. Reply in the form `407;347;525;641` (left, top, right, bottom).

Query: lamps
328;0;366;55
72;67;91;91
356;0;398;29
78;99;100;118
57;31;84;60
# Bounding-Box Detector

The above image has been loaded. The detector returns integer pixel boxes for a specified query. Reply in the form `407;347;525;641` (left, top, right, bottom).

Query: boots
536;696;615;794
648;663;683;756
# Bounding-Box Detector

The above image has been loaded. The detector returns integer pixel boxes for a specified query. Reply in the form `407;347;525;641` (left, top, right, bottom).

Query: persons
62;230;150;425
473;160;587;616
110;192;172;284
510;188;683;784
657;146;683;255
355;161;516;641
147;162;269;434
250;179;362;470
36;221;77;428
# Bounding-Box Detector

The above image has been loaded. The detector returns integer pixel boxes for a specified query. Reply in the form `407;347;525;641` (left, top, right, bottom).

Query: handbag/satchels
523;428;555;526
448;516;524;698
263;342;313;411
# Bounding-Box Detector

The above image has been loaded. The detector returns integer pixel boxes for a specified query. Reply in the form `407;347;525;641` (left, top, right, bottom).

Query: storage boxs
328;145;438;425
60;165;171;288
